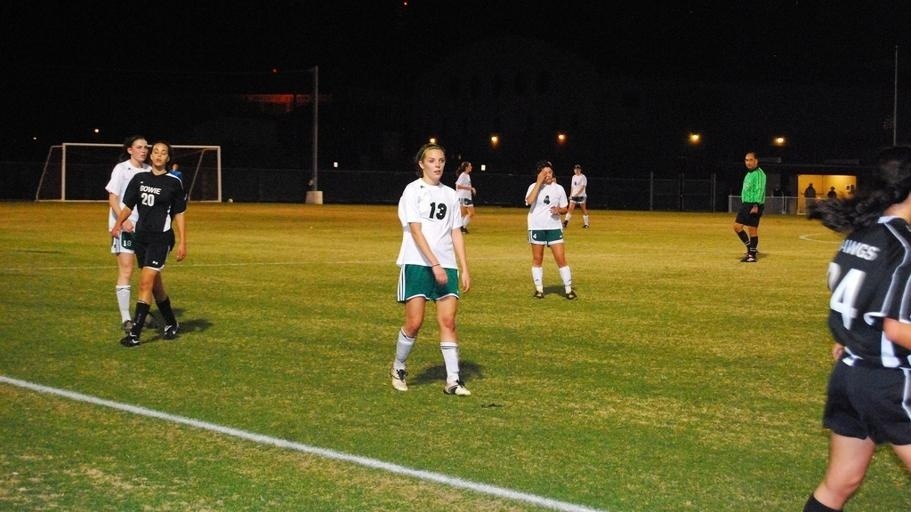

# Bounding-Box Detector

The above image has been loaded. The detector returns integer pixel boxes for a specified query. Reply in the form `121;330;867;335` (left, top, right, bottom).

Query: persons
805;183;818;215
800;142;911;511
563;164;591;230
454;160;477;235
522;157;580;301
772;184;784;215
732;150;768;262
104;135;158;334
168;162;183;184
828;186;838;198
109;140;188;347
388;142;476;397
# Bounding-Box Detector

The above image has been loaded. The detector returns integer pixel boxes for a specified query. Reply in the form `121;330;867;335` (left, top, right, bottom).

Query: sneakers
390;367;408;392
741;249;759;263
533;291;543;299
582;225;589;228
562;220;568;228
565;291;575;299
461;226;469;233
121;312;181;346
444;380;471;396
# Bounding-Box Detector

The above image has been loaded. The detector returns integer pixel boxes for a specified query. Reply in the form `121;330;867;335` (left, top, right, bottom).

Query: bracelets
431;263;441;269
